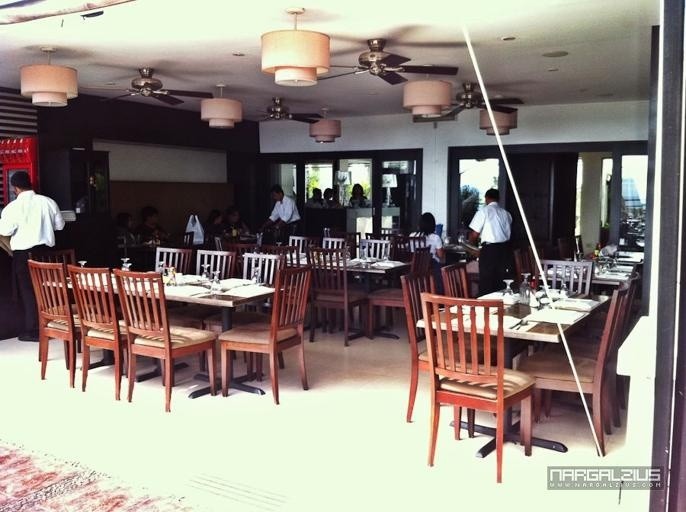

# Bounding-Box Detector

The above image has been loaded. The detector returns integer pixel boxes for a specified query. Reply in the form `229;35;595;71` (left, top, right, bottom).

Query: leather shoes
18;334;38;341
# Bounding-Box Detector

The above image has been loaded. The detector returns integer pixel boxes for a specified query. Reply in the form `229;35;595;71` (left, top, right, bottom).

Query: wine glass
520;273;532;295
122;258;133;272
251;266;261;291
277;243;283;247
211;271;221;294
157;262;165;274
78;260;87;268
201;264;211;284
503;279;514;305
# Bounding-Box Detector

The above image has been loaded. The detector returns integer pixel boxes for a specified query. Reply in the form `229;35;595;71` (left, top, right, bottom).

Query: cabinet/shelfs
42;147;112;268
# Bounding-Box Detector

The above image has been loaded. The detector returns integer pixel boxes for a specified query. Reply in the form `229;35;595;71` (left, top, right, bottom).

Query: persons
257;184;301;236
136;206;168;241
305;188;324;208
469;189;515;293
323;189;334;208
118;213;134;243
0;171;65;341
202;210;223;244
223;206;249;235
408;213;443;294
349;184;367;208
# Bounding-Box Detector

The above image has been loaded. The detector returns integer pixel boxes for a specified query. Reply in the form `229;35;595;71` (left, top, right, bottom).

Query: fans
245;96;324;124
315;38;460;86
437;83;525;119
81;68;212;106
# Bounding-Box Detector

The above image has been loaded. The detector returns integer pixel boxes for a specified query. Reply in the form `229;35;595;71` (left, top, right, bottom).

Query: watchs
275;225;279;229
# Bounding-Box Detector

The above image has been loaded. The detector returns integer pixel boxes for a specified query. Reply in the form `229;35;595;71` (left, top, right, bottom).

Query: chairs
534;258;594;296
513;246;533;284
260;246;311;305
400;269;497;438
440;262;526;358
396;235;426;288
377;227;400;235
358;239;390;260
419;292;537;483
303;245;368;347
26;259;112;388
368;244;431;340
27;248;108;369
516;281;631;456
166;250;237;373
112;269;222;413
565;272;640;411
202;252;286;382
558;235;575;257
328;230;360;247
224;242;259;264
365;233;397;260
287;235;315;254
66;264;160;402
115;247;193;322
217;264;311;405
321;237;349;257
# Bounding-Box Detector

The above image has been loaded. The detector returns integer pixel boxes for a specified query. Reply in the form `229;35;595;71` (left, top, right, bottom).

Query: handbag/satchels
183;215;206;246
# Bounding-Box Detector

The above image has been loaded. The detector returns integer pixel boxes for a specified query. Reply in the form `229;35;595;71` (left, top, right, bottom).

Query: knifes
510;319;523;329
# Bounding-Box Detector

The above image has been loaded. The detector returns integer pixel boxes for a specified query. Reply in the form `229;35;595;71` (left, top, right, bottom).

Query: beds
415;288;612;459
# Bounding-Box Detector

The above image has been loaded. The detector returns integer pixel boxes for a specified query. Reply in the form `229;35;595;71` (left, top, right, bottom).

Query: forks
517;321;527;330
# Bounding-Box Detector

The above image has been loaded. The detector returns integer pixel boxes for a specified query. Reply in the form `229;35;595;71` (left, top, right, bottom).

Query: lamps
19;47;78;107
260;7;330;86
309;108;341;143
199;84;242;128
479;94;517;136
402;64;452;117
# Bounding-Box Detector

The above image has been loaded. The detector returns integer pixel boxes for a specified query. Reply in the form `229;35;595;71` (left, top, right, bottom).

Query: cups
444;237;450;248
458;235;462;246
